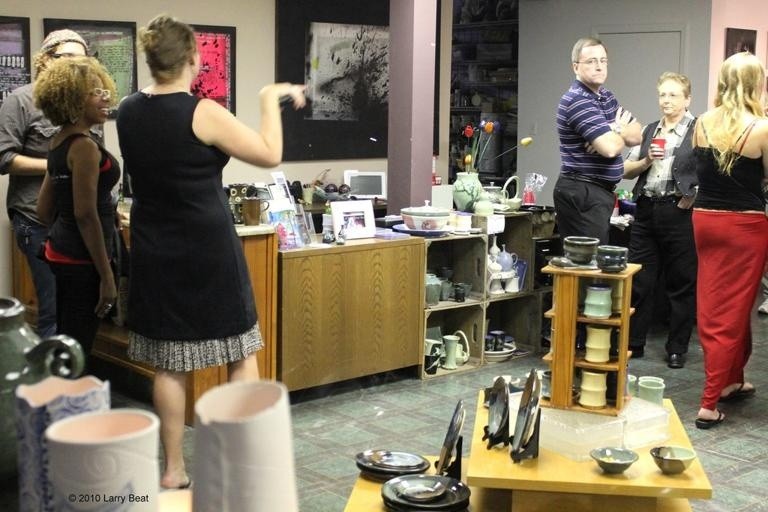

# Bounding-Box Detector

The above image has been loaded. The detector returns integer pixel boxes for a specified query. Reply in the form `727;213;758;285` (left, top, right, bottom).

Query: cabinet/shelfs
423;206;543;379
6;215;280;432
339;375;717;512
533;260;642;419
278;223;426;401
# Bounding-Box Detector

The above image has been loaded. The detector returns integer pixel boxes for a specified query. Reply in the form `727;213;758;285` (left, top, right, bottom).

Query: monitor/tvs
344;172;388;200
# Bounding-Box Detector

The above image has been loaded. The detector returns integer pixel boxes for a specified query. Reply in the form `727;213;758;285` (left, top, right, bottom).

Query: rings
106;303;112;311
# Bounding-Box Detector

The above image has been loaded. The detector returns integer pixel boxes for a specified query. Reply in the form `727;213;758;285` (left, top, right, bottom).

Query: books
258;197;304;249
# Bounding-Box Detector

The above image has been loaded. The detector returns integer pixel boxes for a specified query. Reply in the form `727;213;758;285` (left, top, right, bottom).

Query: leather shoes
667;351;684;368
625;341;645;358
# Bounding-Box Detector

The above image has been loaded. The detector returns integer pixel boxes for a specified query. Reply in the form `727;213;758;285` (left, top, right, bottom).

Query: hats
38;29;88;55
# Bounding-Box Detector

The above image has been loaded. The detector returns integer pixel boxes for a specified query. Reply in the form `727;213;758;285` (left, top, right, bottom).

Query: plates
393;223;453;237
350;368;541;512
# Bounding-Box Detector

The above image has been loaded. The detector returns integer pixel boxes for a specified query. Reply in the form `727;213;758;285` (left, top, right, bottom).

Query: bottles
482;181;502;206
117;183;124;202
289;179;313;205
322;224;346;244
612;192;619;217
426;266;468;374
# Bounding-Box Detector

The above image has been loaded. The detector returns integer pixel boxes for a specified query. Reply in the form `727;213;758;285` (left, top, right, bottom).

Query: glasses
89;87;111;98
575;59;607;65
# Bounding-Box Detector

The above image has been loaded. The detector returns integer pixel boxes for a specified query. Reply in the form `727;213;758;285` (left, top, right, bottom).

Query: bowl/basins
588;448;638;472
503;199;522;212
401;196;450;230
651;446;696;473
520;205;557;238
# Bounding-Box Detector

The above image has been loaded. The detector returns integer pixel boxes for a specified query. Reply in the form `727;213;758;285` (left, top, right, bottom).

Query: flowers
454;118;534;170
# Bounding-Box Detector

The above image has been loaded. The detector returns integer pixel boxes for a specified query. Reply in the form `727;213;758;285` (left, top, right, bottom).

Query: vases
424;272;443;305
42;404;163;512
453;173;484;214
0;288;95;511
10;376;117;511
191;375;301;512
442;328;461;373
321;213;335;237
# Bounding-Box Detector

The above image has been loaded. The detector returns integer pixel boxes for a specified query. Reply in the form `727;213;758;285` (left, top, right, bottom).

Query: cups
452;209;483;235
596;246;627;273
0;296;303;512
486;236;526;361
563;235;600;265
583;284;612;320
228;182;269;226
651;138;665;157
541;371;552;399
585;326;613;363
627;373;668;403
577;370;608;410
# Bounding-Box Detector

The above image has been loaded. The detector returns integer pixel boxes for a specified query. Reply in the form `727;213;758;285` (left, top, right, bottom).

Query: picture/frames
0;14;34;102
39;16;140;121
169;22;238;121
329;197;378;241
272;0;442;166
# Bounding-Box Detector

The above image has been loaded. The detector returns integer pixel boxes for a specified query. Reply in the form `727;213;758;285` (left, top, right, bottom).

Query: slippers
694;407;725;429
717;380;755;401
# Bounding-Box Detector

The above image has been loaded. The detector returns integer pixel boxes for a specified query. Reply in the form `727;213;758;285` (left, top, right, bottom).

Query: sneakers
759;296;767;314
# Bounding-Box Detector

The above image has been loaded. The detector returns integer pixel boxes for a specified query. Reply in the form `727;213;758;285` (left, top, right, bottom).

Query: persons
558;38;644;311
692;50;767;428
36;54;129;364
623;71;700;367
0;28;90;338
759;268;768;314
115;15;309;489
